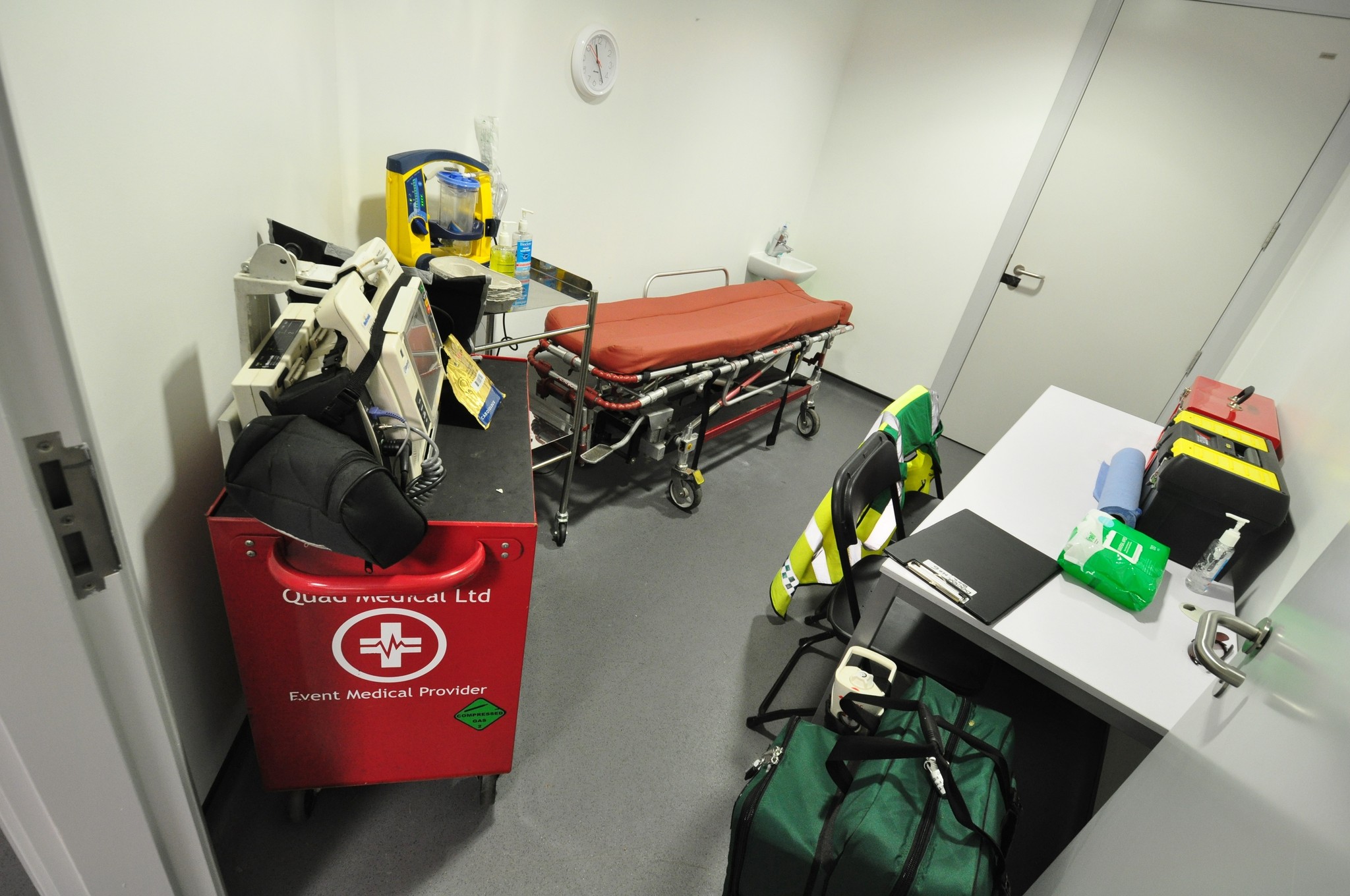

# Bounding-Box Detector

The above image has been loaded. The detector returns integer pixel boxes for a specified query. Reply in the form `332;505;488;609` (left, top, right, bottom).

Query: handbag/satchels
223;412;430;575
715;678;1022;896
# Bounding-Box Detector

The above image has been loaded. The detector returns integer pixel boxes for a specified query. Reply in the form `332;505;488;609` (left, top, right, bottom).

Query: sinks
747;252;817;285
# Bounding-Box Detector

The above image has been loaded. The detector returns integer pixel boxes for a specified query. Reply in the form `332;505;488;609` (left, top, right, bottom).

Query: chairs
797;385;947;638
745;433;981;751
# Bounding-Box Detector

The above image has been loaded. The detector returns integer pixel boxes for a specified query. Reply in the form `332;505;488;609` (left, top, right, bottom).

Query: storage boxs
1129;408;1291;587
1145;372;1283;495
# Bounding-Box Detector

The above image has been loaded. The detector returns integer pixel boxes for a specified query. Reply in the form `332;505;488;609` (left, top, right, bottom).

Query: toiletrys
1186;512;1251;596
490;219;518;281
514;208;535;306
781;222;789;240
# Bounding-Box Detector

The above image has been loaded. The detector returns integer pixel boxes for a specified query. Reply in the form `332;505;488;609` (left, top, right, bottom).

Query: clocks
570;25;625;106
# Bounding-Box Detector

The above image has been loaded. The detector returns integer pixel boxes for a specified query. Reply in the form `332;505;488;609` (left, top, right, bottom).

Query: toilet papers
1092;448;1145;529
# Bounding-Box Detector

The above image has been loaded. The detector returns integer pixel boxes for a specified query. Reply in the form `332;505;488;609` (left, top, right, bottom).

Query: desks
803;380;1245;857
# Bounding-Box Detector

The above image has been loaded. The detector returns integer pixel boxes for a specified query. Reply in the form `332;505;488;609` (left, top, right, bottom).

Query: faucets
767;231;793;257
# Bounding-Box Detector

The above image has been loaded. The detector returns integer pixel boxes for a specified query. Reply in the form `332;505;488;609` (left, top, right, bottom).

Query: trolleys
468;252;601;545
